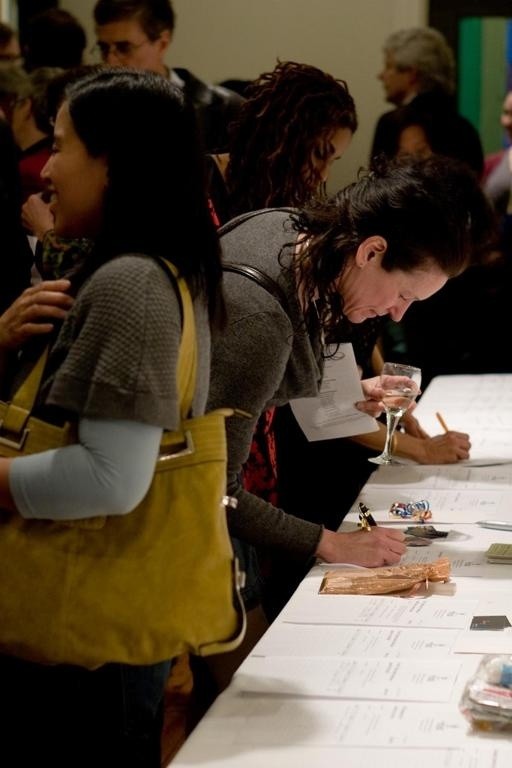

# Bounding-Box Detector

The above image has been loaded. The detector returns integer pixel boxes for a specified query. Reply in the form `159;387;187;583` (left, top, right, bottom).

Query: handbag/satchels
0;257;246;671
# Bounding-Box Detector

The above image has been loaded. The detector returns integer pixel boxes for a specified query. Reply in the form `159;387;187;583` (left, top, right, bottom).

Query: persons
1;3;472;768
369;26;511;401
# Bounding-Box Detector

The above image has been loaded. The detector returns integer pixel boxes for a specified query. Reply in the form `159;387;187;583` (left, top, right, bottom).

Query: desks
165;373;512;768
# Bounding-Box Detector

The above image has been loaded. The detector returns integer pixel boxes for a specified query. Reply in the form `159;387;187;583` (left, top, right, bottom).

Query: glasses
90;42;146;58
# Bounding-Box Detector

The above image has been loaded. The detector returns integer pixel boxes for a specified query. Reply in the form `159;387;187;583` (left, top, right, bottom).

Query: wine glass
366;361;424;469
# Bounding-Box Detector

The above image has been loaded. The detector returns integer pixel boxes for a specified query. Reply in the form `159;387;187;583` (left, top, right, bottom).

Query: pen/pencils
359;503;377;527
437;414;452;433
478;521;512;532
